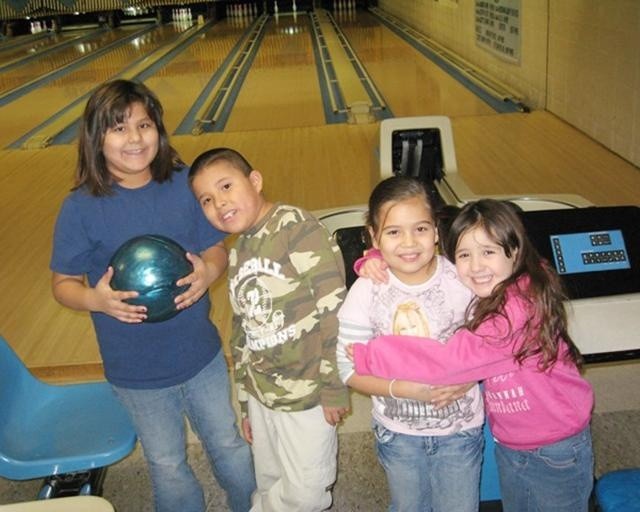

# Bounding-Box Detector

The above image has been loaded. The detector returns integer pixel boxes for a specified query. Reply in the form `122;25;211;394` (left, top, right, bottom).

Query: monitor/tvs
546;227;633;278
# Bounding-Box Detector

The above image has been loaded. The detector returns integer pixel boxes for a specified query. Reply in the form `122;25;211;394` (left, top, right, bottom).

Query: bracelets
388;379;403;400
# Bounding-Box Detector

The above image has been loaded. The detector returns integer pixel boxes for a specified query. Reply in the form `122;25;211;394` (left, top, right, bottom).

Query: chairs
1;495;116;511
0;334;136;481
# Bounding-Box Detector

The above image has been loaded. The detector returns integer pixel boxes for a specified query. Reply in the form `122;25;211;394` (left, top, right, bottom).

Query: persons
392;301;431;338
344;200;597;511
336;174;487;510
51;80;258;511
188;148;353;512
